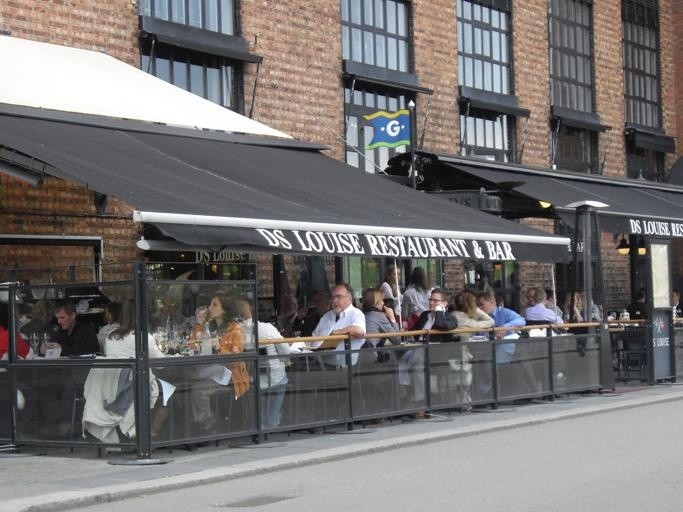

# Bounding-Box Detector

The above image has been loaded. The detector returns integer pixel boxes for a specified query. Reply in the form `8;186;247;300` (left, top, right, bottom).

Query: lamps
633;234;647;256
612;232;630;256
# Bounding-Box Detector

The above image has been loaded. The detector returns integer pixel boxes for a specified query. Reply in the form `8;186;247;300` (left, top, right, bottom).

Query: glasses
428;298;445;303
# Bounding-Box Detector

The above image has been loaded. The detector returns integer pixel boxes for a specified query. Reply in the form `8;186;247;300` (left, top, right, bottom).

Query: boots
143;406;173;454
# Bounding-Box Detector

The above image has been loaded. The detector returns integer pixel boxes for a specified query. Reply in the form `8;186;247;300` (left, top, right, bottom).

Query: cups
201;337;212;355
156;338;167;353
610;311;616;320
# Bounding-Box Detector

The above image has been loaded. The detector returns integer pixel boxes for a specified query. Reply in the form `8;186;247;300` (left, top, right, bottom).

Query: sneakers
412;402;428;418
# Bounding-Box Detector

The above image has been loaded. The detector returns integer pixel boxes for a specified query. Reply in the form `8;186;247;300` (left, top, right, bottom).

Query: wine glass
27;331;51;358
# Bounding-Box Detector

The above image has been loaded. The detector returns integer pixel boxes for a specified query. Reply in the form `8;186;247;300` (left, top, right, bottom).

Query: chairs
0;313;645;454
